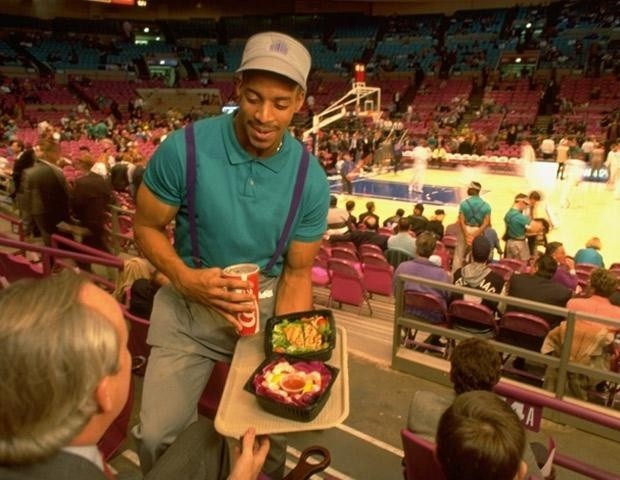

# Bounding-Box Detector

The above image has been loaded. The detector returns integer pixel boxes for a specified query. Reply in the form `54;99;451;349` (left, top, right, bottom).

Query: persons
431;391;530;480
128;30;331;479
406;338;540;479
1;0;620;392
0;268;271;479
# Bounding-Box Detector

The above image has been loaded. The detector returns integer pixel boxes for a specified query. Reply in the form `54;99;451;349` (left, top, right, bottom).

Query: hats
515;196;531;205
465;182;480;190
236;31;313;90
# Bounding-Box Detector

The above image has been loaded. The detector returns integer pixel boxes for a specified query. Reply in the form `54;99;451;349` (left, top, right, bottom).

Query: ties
103;463;117;480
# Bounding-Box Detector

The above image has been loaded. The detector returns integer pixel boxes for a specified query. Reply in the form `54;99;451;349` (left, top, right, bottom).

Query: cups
221;262;261;338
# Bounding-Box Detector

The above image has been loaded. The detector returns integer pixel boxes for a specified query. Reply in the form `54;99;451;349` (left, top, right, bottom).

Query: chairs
402;289;448;358
583;329;619;409
496;310;551;384
398;430;449;480
444;300;500;355
